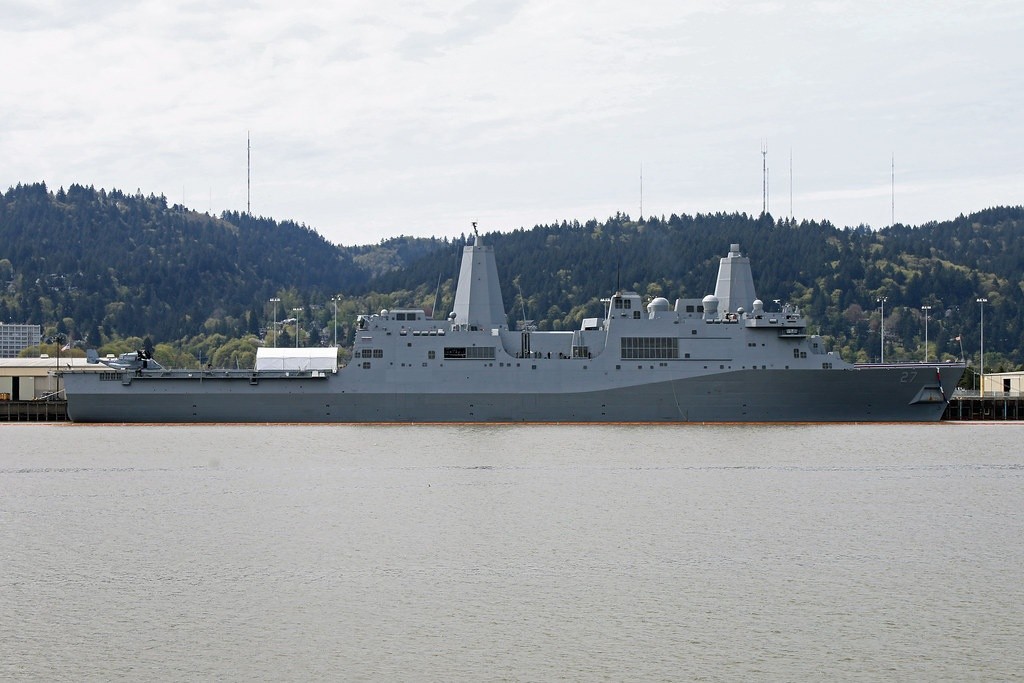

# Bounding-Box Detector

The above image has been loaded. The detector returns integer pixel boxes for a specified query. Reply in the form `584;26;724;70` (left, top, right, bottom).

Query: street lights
269;298;281;348
921;305;932;362
293;307;302;348
331;295;341;347
876;296;887;363
976;298;988;376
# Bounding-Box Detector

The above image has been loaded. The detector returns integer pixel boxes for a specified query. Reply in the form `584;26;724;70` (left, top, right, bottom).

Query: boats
46;218;978;426
